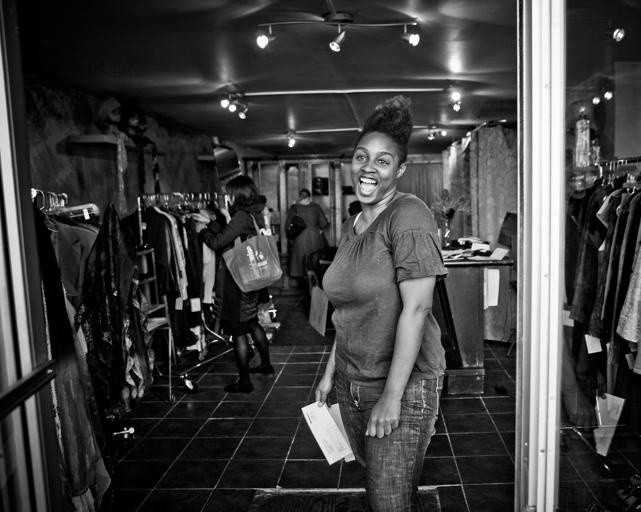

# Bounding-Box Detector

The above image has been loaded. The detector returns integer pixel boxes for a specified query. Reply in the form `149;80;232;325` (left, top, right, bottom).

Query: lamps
256;13;420;53
447;85;465;113
607;23;626;43
220;94;251;119
429;126;447;142
287;133;297;148
576;80;616;115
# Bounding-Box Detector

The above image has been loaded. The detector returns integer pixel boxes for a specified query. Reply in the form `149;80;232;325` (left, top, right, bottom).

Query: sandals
224;362;274;393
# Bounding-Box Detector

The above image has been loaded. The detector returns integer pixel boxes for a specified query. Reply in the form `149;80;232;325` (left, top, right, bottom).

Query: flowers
431;189;472;220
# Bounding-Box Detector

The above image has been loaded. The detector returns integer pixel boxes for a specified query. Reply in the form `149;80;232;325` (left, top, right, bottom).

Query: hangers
31;187;101;227
139;189;225;214
591;160;641;188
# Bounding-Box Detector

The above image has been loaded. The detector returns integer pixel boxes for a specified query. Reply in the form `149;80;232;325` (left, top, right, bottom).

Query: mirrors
535;0;640;512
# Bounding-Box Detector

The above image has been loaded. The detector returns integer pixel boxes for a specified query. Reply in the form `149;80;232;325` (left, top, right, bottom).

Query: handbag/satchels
222;213;283;294
286;205;305;240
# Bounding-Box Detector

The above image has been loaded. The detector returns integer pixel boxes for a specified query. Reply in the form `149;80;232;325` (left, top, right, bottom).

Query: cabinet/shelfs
128;248;178;367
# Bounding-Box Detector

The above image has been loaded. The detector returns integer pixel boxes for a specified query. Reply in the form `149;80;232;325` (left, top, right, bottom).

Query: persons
285;185;328;294
190;175;283;393
314;94;449;512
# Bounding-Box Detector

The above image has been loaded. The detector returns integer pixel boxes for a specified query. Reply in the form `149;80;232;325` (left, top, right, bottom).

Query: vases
442;219;452;247
